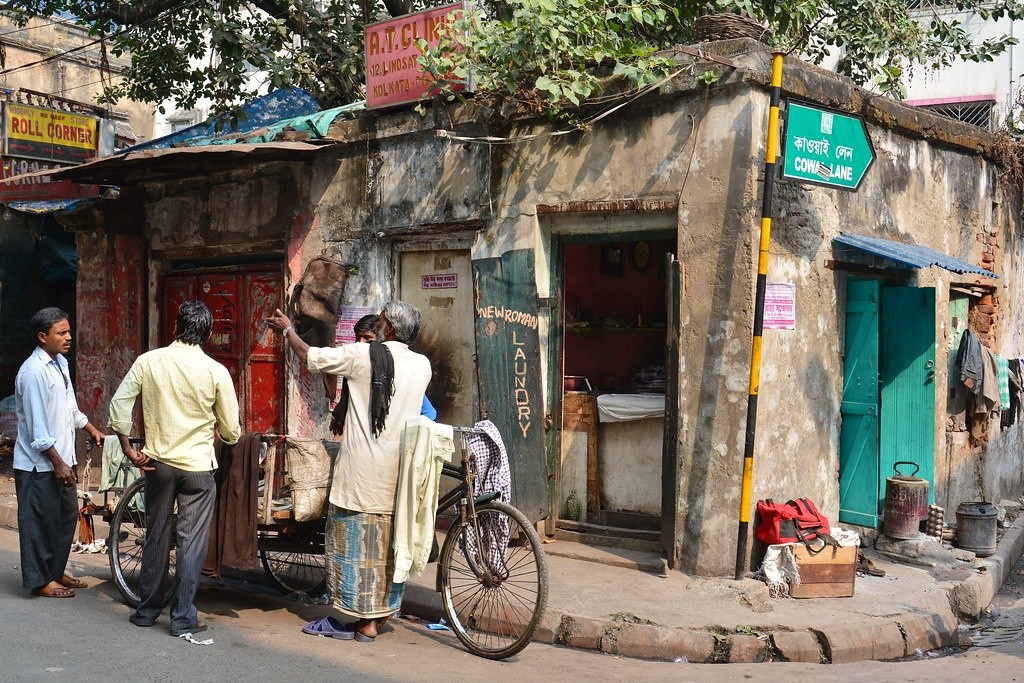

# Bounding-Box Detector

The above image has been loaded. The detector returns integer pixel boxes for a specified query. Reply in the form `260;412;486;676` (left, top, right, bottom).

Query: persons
102;300;242;638
9;306;108;597
262;300;435;639
326;314;438;424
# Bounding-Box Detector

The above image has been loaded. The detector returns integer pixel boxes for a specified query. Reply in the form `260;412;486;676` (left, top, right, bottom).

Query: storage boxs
788;544;857;598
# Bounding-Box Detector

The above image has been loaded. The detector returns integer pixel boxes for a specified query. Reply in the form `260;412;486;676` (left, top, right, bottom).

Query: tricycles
79;423;549;659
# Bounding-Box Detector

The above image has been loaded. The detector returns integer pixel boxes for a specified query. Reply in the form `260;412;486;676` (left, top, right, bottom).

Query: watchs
278;326;294;339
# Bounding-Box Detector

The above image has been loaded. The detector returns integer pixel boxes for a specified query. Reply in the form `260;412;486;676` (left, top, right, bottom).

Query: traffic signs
781;97;876;194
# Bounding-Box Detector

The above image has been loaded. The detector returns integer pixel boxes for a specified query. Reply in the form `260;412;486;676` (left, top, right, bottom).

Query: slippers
55;574;88;588
302;615;355;640
171;620;208;637
346;622;375;642
128;613;155;626
37;580;75;598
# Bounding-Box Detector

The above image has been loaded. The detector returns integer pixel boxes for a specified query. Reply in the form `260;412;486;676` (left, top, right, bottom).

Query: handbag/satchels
755;497;831;552
